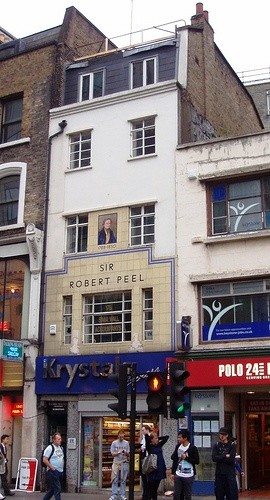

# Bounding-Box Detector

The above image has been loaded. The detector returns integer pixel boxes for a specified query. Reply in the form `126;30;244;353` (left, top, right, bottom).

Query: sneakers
109;495;118;500
119;495;127;500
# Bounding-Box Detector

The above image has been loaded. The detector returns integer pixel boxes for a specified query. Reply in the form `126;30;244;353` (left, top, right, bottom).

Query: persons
109;429;130;500
231;437;244;478
141;429;169;500
171;429;200;500
0;434;16;496
42;432;64;500
99;218;116;245
139;425;152;476
212;427;239;500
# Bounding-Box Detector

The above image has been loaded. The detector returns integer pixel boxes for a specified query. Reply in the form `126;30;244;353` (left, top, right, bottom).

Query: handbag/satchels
141;450;157;475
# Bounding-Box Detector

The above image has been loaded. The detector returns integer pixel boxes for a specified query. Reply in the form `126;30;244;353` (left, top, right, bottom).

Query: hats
219;428;229;434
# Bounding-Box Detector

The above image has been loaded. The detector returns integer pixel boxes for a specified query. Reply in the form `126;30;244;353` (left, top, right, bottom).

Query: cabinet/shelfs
82;416;155;487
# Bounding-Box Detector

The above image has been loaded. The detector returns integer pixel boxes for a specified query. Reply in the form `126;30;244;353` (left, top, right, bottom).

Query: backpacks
41;444;54;467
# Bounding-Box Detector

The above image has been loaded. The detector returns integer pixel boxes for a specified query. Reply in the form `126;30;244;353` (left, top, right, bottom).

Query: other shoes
7;493;15;496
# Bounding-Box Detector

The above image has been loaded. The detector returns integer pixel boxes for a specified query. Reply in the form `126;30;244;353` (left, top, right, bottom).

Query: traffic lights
107;362;191;421
